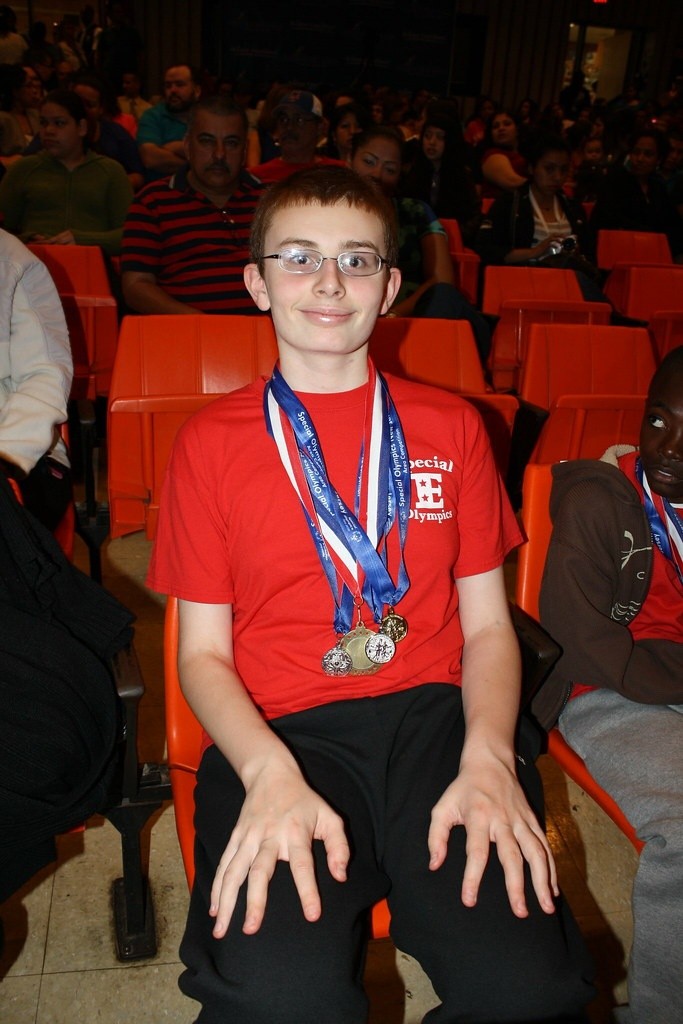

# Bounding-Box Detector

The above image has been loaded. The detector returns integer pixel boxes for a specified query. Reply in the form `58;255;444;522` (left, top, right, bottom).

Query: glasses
256;247;390;277
21;81;34;89
276;115;317;128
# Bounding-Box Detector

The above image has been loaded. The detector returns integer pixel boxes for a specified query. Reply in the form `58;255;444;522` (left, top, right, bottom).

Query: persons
145;162;587;1024
539;343;683;1024
0;0;683;542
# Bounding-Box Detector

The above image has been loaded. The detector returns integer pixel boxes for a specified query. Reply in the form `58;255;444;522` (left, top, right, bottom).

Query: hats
270;89;324;123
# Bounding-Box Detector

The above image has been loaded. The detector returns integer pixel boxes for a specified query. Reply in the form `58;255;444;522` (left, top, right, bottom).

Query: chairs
0;208;683;959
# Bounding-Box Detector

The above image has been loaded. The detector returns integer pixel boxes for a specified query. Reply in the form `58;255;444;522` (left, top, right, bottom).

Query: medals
322;615;408;678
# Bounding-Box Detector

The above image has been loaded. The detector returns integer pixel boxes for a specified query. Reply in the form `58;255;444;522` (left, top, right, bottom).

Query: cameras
546;236;575;258
